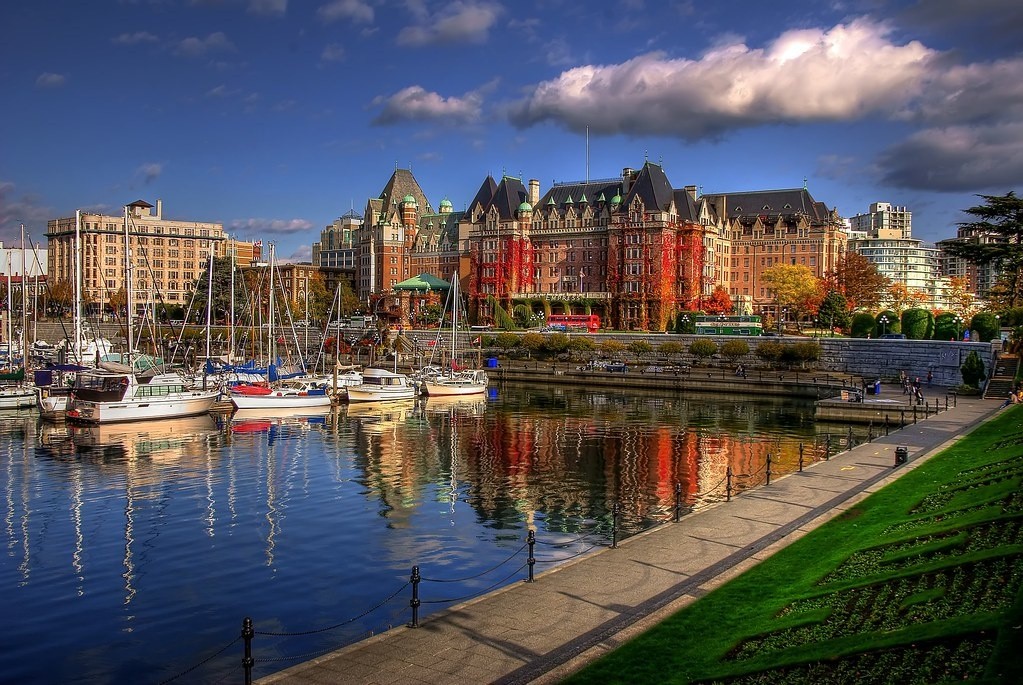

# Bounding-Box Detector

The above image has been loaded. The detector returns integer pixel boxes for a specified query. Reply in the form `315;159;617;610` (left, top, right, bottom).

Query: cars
328;321;344;328
762;331;777;336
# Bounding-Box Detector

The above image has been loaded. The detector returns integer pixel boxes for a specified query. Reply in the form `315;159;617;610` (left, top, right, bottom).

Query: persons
1010;337;1015;349
1004;390;1023;406
1003;338;1008;352
899;370;933;395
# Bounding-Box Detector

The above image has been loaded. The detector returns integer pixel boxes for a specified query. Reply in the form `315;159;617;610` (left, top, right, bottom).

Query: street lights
879;315;890;334
953;313;966;341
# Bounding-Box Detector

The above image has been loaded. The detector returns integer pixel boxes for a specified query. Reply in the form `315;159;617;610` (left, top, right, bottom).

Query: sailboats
0;205;467;425
424;269;489;398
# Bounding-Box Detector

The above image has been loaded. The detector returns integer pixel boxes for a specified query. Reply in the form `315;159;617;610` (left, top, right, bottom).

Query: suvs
294;319;306;327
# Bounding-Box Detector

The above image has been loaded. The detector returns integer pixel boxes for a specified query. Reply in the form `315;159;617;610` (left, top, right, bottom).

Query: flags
472;336;480;343
278;337;282;343
253;240;262;247
428;341;435;345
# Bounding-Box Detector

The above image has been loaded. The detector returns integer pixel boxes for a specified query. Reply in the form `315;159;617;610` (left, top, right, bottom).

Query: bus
692;314;763;338
546;312;602;332
351;316;371;329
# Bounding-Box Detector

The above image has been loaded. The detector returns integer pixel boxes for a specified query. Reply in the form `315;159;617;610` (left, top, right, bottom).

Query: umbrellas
391;273;454;330
